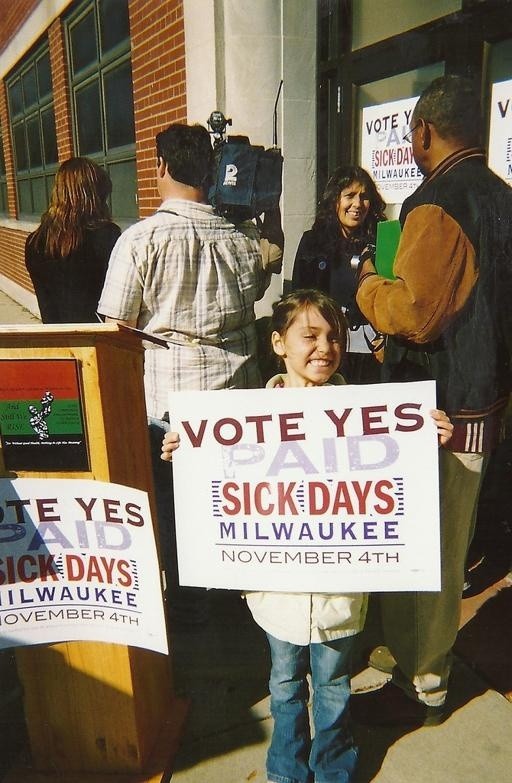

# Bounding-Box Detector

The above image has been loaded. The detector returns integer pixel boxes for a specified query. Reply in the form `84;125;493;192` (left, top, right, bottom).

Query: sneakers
350;683;444;727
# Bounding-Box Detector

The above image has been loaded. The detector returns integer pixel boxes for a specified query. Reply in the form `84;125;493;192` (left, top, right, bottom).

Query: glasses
402;125;421;143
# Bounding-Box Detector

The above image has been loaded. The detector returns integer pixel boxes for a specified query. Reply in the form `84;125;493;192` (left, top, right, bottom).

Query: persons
292;164;388;384
28;392;54;441
354;74;512;728
98;124;283;629
161;289;454;783
25;158;120;323
